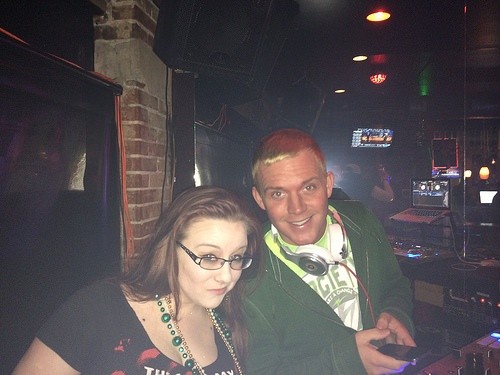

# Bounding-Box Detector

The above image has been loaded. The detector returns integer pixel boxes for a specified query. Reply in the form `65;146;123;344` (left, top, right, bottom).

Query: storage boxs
415;281;448;307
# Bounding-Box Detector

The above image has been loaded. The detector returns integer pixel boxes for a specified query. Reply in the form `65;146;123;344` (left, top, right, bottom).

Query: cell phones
377;343;420;361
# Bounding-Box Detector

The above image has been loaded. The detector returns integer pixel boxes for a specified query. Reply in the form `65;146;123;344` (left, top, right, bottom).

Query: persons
13;185;264;375
221;128;420;375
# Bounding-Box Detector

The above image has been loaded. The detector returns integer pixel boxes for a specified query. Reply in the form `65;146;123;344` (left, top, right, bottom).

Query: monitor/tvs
478;190;498;204
352;128;394;148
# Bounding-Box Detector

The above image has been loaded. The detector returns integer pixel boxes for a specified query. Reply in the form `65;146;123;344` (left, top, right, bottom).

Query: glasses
175;241;254;270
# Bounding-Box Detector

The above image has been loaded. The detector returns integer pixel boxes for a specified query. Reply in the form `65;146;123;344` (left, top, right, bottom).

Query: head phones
271;204;348;276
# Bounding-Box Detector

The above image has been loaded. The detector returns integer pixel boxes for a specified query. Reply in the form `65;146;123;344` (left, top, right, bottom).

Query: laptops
390;176;452;224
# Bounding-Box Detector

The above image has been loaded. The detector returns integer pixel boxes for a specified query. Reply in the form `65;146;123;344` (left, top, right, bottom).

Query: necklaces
154;289;245;375
